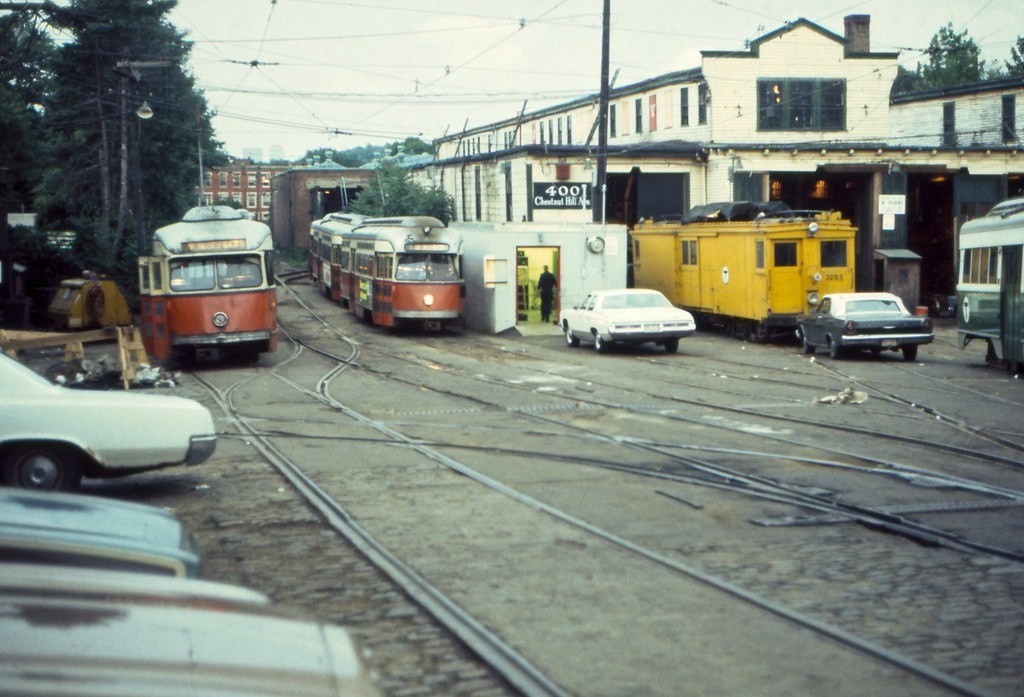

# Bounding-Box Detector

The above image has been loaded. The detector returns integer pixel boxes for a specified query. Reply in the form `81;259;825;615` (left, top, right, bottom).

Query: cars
796;290;935;361
560;290;696;354
0;356;215;495
1;486;384;697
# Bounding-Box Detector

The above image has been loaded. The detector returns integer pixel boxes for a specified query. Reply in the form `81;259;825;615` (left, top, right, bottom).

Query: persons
538;265;557;322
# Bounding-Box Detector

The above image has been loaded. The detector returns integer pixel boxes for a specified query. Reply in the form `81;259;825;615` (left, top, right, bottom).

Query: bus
307;214;466;334
956;200;1023;373
628;201;857;342
138;202;279;363
